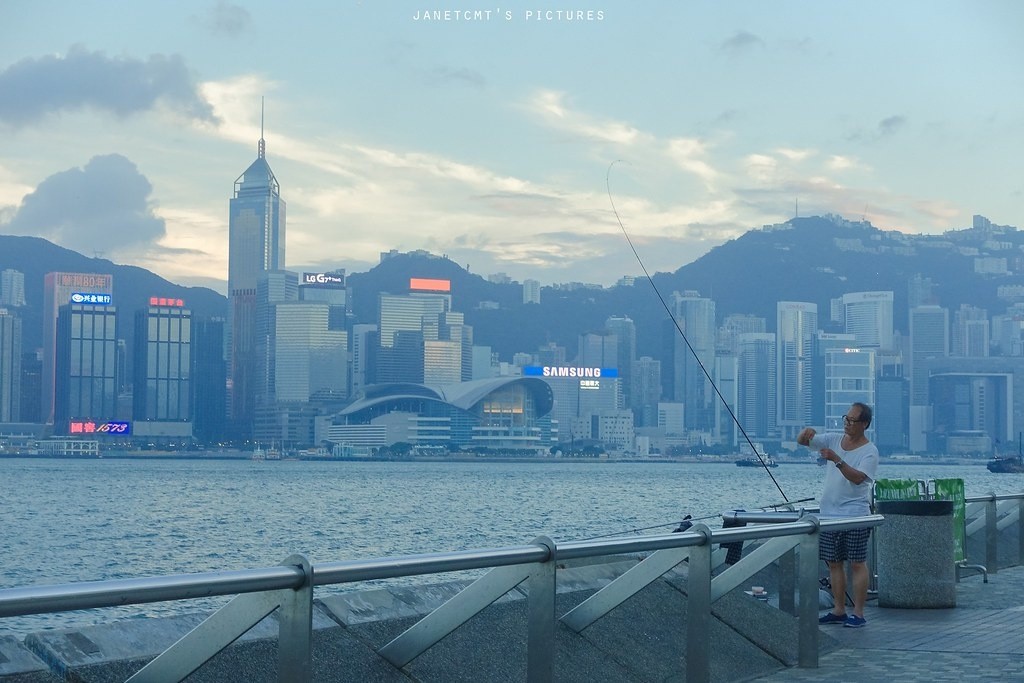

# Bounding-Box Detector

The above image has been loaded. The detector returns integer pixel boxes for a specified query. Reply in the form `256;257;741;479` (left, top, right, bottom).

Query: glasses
842;415;862;425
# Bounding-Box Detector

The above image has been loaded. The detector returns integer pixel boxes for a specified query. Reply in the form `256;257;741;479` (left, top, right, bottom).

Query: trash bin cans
875;499;956;608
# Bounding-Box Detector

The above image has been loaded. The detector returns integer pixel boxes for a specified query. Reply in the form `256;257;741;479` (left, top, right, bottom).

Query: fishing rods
605;159;857;609
572;496;816;541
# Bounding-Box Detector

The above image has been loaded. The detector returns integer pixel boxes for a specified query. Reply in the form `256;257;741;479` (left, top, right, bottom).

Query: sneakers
819;612;848;625
844;614;867;627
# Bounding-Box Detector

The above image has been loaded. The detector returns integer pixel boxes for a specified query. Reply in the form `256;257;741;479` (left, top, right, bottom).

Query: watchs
836;460;846;468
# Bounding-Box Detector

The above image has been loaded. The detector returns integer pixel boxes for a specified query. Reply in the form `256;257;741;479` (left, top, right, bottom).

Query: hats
744;586;767;596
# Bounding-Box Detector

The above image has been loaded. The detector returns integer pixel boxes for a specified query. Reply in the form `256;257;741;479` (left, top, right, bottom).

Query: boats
985;431;1024;475
734;448;779;468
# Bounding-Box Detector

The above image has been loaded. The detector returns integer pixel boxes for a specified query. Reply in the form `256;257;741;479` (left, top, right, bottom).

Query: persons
795;403;882;627
